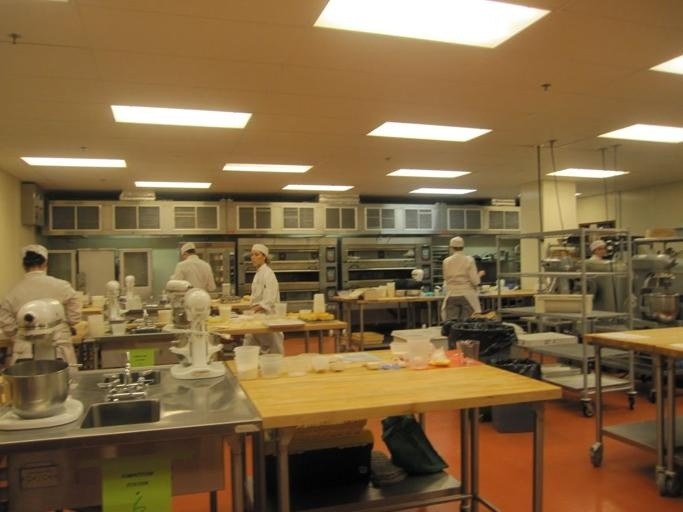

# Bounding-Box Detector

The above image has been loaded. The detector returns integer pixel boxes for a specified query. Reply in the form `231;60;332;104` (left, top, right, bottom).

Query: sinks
127;328;162;334
80;369;162;429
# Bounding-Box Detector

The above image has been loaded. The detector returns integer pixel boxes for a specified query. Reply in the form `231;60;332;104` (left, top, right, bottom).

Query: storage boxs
533;294;594;315
259;426;373;506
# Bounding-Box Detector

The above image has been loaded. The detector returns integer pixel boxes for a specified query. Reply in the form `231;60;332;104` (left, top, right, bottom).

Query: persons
1;241;82;370
239;242;288;362
590;239;611;261
171;241;216;303
443;237;484;323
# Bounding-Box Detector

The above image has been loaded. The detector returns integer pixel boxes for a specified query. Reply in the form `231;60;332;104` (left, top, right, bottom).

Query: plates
264;318;306;328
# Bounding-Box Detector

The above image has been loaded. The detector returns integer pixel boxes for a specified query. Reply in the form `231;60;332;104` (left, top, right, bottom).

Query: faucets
97;350;154;403
142;308;150;327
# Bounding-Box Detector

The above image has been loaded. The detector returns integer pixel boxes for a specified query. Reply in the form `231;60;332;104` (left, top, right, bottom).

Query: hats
181;243;195;255
22;244;48;260
252;244;269;256
590;240;606;252
450;236;464;247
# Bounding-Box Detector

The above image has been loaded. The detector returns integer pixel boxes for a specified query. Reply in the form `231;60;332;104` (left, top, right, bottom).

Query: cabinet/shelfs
494;226;635;416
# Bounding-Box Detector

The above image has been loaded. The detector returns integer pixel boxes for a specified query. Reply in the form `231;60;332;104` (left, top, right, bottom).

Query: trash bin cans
491;358;541;432
441;318;518;420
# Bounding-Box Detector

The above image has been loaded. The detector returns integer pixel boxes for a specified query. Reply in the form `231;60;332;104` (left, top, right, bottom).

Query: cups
455;340;479;365
233;345;257;380
92;296;104;307
158;310;171;321
273;301;287;317
222;283;230;298
287;356;307;376
87;313;104;337
259;351;282;378
312;293;325;314
218;305;230;320
111;323;126;335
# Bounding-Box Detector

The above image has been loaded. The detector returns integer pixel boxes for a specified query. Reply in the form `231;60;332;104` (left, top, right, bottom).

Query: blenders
123;275;142;310
168;285;225;380
164;278;192;328
104;280;125;323
0;298;84;431
